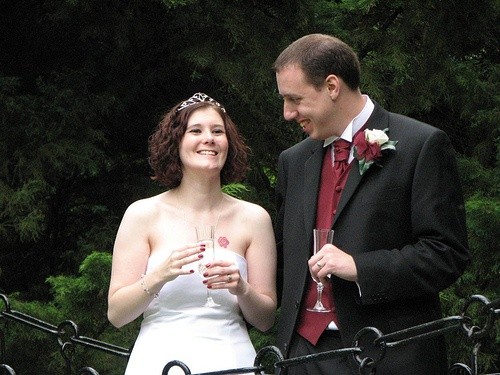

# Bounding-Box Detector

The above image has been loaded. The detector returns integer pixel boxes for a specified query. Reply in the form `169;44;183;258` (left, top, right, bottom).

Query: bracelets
140;276;158;298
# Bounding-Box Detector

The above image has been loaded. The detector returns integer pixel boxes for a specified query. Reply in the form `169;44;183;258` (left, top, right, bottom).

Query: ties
333;138;353;181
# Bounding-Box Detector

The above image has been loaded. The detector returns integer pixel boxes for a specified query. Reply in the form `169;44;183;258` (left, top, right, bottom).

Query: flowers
354;128;398;176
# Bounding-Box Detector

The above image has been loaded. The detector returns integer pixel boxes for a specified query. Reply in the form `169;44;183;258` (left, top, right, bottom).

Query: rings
316;262;322;268
227;274;232;281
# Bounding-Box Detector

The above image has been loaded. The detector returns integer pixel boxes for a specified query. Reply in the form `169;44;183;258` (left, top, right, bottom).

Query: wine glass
306;229;335;313
194;225;223;307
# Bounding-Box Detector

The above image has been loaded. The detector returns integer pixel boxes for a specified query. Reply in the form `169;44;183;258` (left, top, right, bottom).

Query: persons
107;91;277;375
243;34;469;375
